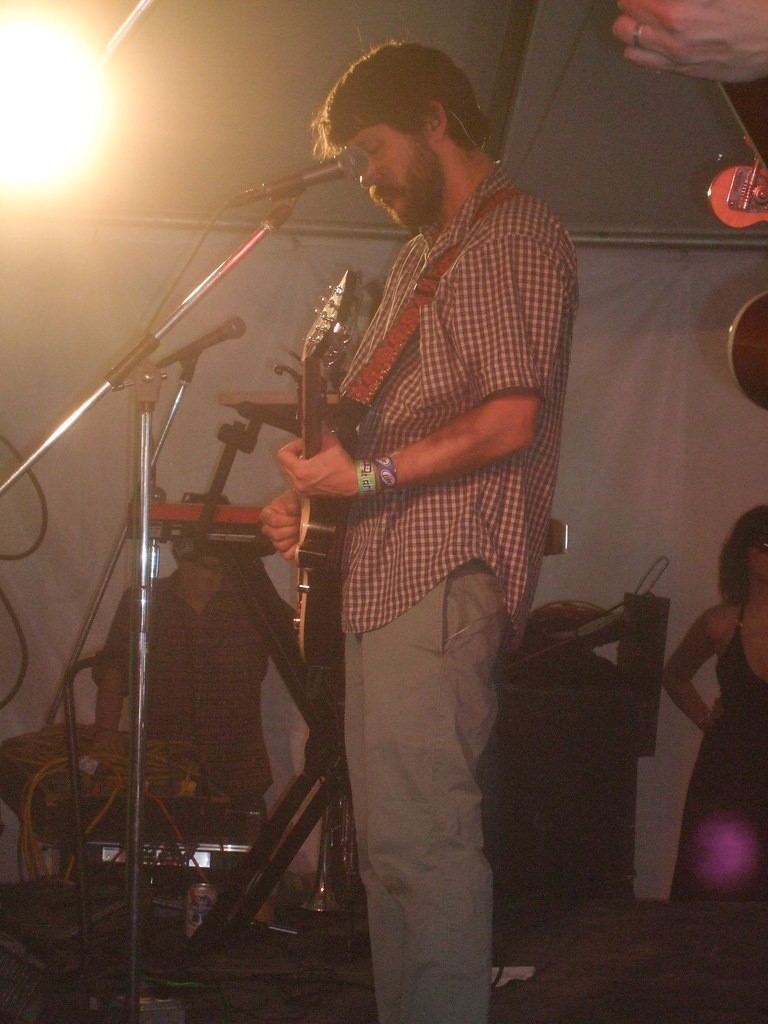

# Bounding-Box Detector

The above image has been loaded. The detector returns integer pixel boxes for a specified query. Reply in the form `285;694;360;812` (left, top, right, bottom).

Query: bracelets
355;455;398;500
697;710;713;726
96;724;118;732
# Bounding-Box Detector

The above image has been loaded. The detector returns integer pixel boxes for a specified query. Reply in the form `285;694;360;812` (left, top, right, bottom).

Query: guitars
704;73;768;415
286;262;363;673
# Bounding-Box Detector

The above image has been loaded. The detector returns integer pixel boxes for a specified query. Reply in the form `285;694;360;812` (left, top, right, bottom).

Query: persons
91;490;274;824
659;502;768;902
259;38;581;1024
609;0;768;83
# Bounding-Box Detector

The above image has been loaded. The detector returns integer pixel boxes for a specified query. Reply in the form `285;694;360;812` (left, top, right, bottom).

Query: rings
631;20;646;49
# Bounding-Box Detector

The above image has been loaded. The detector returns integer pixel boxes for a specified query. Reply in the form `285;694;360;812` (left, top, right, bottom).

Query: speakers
470;684;636;931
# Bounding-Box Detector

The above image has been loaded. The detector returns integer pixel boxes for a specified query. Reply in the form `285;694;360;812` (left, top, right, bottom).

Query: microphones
225;146;369;208
155;317;246;369
